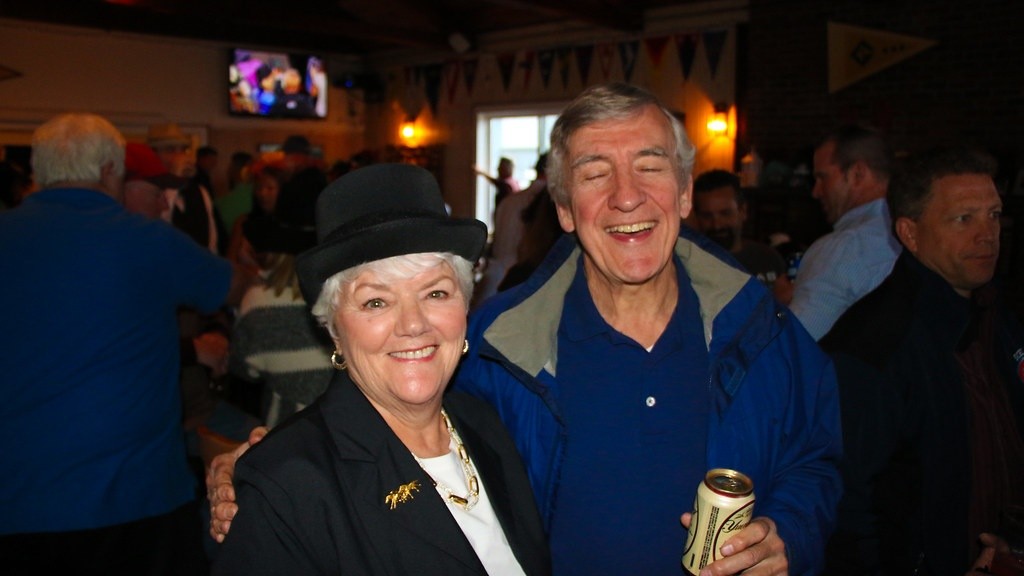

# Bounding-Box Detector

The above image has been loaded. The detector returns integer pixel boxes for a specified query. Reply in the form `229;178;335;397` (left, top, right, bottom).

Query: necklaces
412;409;479;510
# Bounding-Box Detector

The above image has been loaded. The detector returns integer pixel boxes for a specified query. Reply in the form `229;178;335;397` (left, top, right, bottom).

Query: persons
0;112;237;576
205;82;849;576
0;122;565;456
775;124;903;342
205;162;551;576
695;170;782;294
815;134;1024;576
236;168;341;434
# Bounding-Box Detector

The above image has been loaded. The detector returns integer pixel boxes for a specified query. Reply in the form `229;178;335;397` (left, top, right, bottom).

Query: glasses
159;141;190;155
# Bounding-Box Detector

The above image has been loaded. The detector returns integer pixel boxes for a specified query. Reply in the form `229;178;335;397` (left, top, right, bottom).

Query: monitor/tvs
225;45;329;118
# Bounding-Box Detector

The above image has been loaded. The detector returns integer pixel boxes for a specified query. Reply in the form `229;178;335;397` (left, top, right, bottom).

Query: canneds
682;469;755;576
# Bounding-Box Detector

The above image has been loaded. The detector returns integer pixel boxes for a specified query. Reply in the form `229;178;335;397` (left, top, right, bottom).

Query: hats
275;134;315;156
297;161;489;319
148;121;193;146
